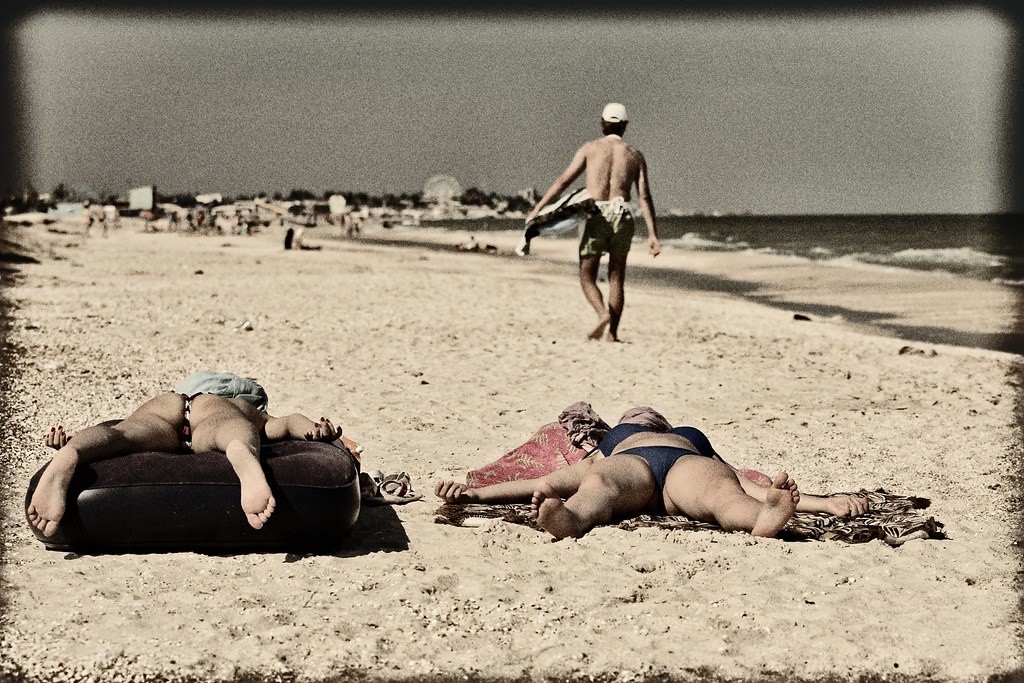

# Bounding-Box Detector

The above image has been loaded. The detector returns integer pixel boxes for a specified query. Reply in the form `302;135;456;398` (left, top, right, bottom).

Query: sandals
359;470;422;504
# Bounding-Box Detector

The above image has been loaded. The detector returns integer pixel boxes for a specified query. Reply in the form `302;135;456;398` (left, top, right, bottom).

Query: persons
82;203;497;252
525;103;661;341
28;372;341;536
436;403;868;539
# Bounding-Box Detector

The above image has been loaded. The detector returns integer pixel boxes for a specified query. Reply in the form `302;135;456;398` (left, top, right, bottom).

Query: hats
601;103;628;123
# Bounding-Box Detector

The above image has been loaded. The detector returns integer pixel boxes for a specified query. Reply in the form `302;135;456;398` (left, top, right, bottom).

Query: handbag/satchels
173;370;268;408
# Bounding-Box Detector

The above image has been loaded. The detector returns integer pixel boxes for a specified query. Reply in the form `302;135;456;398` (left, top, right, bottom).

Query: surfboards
524;184;594;243
